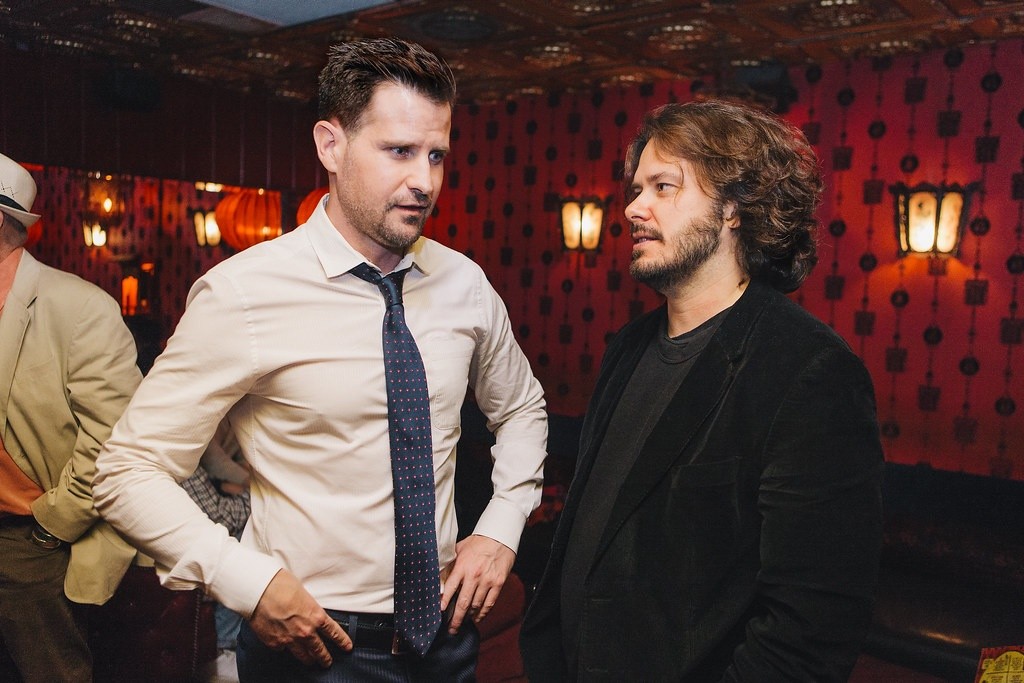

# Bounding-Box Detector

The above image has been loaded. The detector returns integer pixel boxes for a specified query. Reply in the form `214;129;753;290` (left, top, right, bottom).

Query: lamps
556;193;615;254
190;204;224;249
82;217;109;248
888;180;971;258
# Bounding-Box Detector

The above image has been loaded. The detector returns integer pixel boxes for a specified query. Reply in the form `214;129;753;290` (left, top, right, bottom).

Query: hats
0;153;42;227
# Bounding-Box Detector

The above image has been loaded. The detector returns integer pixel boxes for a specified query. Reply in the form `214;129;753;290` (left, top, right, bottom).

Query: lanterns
214;189;282;251
296;187;331;227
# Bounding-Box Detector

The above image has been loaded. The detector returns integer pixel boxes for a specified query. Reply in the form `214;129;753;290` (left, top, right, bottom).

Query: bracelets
32;526;62;548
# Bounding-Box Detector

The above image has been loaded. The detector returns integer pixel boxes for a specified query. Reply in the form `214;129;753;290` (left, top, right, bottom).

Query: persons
519;100;887;683
0;154;146;683
91;38;549;682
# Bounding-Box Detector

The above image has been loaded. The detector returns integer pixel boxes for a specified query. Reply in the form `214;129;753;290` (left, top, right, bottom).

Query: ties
350;264;443;658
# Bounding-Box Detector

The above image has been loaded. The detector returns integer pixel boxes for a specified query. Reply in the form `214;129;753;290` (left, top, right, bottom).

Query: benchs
454;399;1024;683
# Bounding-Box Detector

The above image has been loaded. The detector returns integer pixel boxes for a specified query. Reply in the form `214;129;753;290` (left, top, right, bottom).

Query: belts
334;621;449;649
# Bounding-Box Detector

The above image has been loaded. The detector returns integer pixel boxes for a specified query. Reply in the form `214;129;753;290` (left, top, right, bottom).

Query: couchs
89;565;523;683
